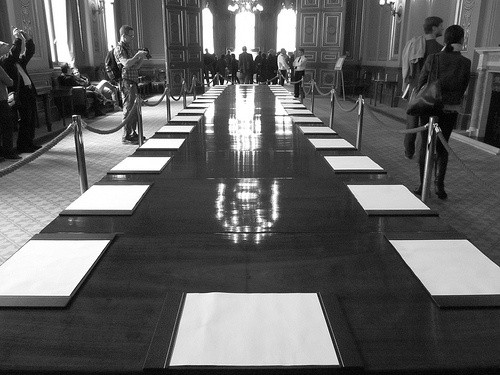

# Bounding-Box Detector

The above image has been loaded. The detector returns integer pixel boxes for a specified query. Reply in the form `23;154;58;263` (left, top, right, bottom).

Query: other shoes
122;134;146;145
4;153;22;159
18;144;42;153
434;180;447;198
413;187;431;197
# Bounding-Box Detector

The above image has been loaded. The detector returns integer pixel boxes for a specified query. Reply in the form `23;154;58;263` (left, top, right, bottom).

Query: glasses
127;34;134;38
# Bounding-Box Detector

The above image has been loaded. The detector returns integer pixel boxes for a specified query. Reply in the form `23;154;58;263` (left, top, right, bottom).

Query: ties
295;57;301;69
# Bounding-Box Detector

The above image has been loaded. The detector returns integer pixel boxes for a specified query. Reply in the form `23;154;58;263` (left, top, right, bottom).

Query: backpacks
104;44;128;85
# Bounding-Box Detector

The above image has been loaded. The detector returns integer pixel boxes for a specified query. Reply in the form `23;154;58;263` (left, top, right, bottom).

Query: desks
373;79;398;107
34;86;54;133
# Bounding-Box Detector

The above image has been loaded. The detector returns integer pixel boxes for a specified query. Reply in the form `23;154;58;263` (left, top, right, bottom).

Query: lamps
379;0;402;17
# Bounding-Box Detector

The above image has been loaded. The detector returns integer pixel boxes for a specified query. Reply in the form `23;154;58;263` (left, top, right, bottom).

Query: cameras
142;47;152;60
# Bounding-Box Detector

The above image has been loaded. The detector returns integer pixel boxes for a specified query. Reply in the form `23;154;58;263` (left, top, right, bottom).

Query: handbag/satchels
406;54;444;115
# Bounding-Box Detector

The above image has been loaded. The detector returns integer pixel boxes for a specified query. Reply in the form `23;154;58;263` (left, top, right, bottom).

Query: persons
402;16;444;158
58;62;112;117
414;25;472;199
0;28;42;153
293;48;308;99
203;46;295;87
0;66;22;164
113;25;148;144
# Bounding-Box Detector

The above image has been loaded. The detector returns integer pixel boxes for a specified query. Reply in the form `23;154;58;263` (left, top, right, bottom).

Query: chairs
52;68;166;131
